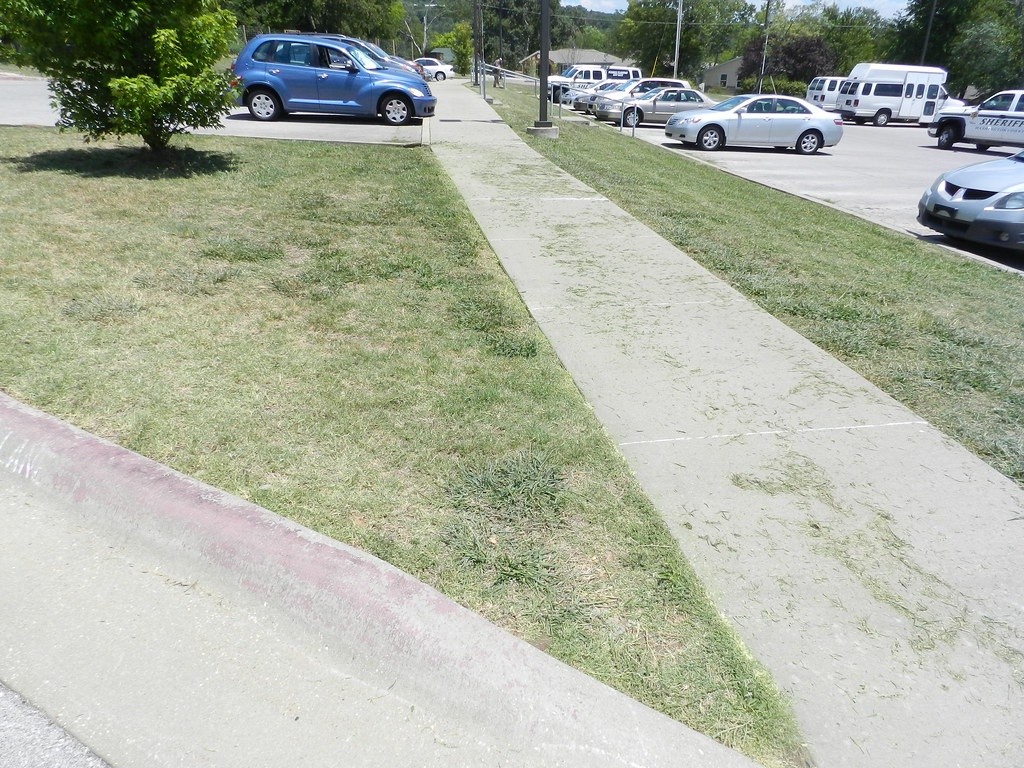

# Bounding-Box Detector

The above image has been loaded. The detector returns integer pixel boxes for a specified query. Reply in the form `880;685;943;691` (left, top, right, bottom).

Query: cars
227;29;438;126
596;86;733;129
664;93;845;157
563;79;627;105
411;57;457;82
574;82;624;115
587;78;692;119
916;149;1024;253
927;89;1024;152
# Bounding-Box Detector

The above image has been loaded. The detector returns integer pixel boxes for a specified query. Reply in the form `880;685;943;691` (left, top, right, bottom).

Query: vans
832;62;967;128
805;75;848;112
547;64;643;103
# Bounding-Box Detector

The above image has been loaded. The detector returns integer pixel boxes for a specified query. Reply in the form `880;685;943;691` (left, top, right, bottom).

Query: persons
491;58;502;87
